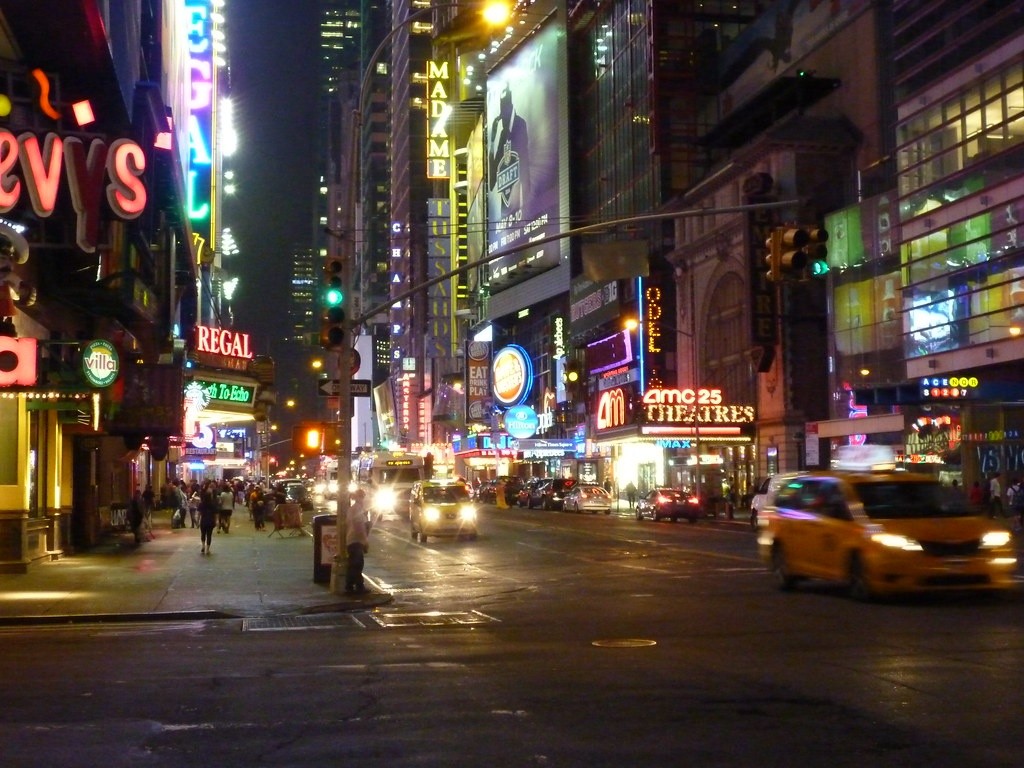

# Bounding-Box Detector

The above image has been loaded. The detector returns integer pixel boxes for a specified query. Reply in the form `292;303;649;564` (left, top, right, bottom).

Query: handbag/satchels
360;522;372;553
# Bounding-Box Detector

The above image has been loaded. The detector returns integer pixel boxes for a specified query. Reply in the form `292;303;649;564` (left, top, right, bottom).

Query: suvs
462;476;541;508
528;477;580;512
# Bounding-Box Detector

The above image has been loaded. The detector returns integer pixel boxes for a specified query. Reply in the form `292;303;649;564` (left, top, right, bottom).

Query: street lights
348;0;513;344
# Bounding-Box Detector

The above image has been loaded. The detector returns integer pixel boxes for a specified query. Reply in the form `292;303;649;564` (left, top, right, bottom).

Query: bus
356;449;427;523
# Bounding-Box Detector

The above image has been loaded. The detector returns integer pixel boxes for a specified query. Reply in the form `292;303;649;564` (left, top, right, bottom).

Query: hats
256;485;260;488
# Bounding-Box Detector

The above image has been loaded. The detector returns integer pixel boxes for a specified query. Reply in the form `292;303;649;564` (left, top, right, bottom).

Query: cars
750;474;784;532
636;487;704;525
756;468;1024;606
562;484;613;515
270;478;314;512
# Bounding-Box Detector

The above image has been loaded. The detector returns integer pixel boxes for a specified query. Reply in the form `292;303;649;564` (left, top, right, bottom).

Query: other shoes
357;586;371;593
346;588;358;598
201;547;205;552
207;551;210;555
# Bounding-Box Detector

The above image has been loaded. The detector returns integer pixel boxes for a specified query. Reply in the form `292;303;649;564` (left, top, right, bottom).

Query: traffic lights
301;421;328;459
316;254;348;354
778;220;830;284
562;361;569;392
566;358;580;392
765;231;782;288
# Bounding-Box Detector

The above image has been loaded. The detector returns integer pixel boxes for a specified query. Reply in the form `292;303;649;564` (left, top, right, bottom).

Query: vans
324;461;358;500
408;478;479;544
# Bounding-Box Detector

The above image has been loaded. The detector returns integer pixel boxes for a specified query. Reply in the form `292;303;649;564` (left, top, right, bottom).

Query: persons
343;490;371;594
129;477;741;554
954;472;1024;514
489;89;531;258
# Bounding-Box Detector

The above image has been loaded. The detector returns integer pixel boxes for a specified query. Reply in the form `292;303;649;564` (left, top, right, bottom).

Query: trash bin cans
312;514;363;587
